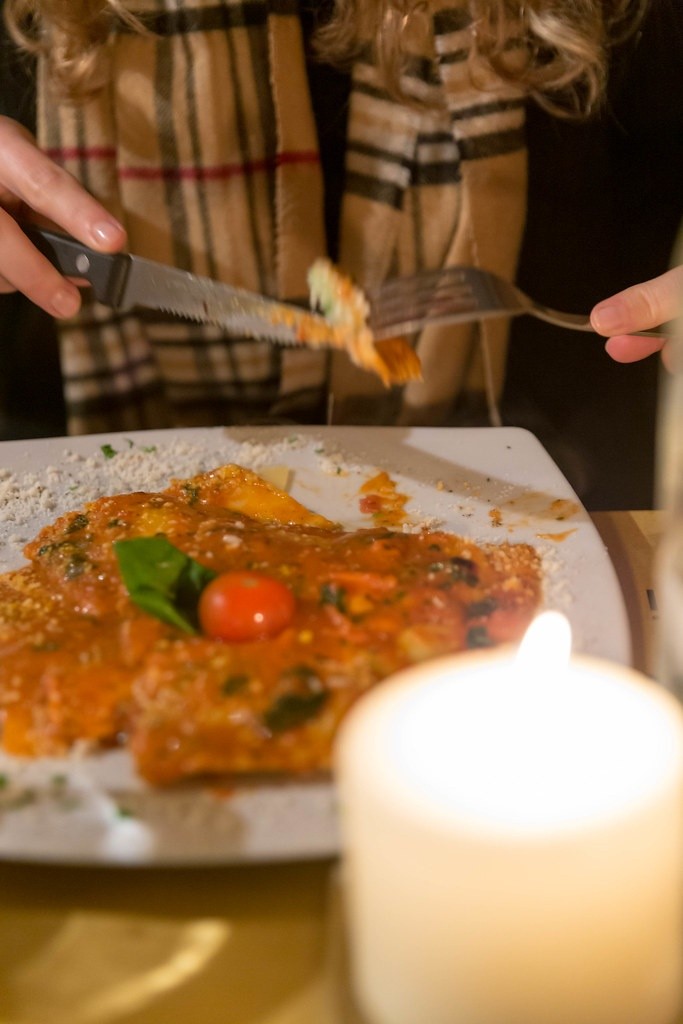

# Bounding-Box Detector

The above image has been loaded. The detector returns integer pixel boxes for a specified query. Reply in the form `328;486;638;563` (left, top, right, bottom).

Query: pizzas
1;463;337;757
308;256;426;384
109;513;539;776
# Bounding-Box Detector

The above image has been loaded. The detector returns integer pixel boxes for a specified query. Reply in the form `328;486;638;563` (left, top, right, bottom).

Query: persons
0;0;683;511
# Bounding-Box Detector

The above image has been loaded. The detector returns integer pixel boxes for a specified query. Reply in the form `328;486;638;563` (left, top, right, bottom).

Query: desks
0;508;659;1024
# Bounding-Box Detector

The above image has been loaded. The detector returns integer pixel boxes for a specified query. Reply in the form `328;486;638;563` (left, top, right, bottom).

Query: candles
338;604;683;1024
